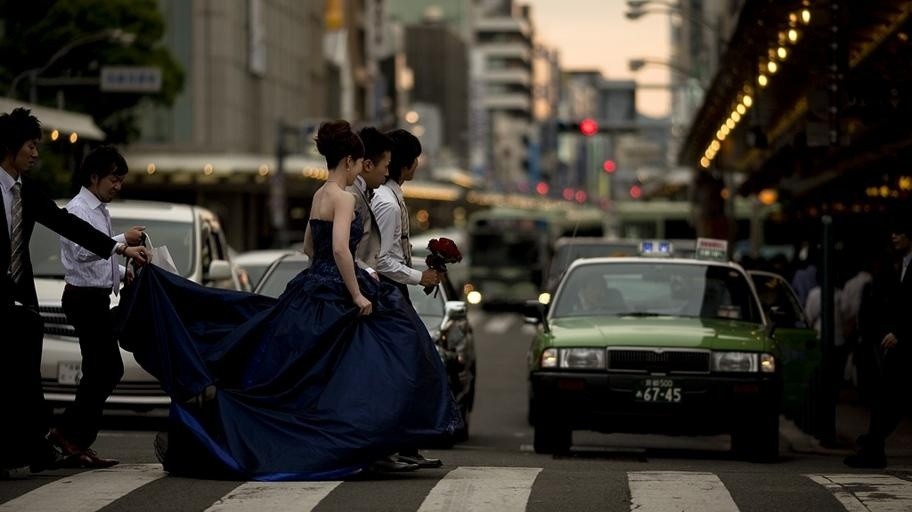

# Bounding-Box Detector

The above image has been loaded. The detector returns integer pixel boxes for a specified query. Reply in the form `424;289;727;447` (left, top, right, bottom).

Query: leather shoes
382;457;441;471
843;453;887;468
30;432;119;472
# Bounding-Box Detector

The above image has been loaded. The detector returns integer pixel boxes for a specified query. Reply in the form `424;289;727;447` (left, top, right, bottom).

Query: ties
400;201;412;267
11;183;23;282
98;205;119;297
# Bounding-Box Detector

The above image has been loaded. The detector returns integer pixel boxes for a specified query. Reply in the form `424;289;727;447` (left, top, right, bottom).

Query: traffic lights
556;120;598;135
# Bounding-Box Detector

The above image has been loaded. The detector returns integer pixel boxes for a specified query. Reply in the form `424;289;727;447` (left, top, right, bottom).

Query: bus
462;202;769;315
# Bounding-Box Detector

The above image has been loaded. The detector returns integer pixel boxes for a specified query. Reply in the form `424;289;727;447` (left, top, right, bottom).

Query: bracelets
116;243;125;255
122;246;128;257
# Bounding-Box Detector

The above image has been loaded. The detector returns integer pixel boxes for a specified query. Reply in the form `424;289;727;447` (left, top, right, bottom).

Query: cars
246;246;485;432
524;237;787;459
9;188;239;428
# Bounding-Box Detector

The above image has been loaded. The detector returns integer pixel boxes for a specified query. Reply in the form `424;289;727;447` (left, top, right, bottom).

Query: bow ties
366;184;375;203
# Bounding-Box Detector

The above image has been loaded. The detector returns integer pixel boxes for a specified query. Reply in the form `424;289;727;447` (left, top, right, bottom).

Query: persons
48;142;149;470
342;125;420;477
557;273;624;318
0;106;153;483
725;216;912;471
300;118;392;476
643;271;718;315
366;128;444;471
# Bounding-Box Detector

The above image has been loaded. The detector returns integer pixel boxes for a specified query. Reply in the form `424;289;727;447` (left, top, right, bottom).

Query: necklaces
326;178;345;190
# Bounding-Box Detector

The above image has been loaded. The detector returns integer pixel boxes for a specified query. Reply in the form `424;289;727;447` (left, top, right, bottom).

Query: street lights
8;25;137;109
625;0;728;86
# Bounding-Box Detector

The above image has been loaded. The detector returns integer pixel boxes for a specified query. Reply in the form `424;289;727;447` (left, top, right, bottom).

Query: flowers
423;236;463;295
